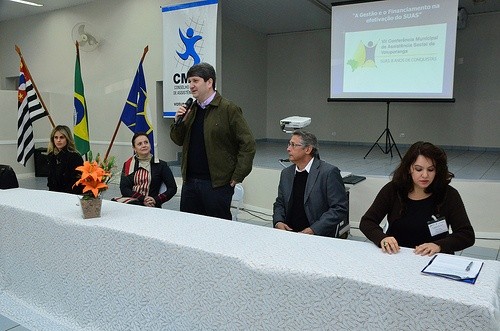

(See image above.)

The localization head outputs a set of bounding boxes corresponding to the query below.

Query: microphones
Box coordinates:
[173,98,193,126]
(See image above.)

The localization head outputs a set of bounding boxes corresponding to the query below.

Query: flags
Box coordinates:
[103,45,155,164]
[14,44,55,168]
[73,40,90,161]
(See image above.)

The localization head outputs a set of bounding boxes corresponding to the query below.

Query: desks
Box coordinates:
[0,188,500,331]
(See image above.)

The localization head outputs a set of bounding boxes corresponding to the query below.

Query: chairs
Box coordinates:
[0,165,19,190]
[230,184,245,222]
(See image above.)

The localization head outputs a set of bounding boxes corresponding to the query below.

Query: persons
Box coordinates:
[170,63,256,221]
[273,130,349,237]
[359,141,475,257]
[111,132,177,208]
[47,125,85,195]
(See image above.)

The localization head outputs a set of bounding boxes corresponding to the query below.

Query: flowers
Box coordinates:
[71,150,118,200]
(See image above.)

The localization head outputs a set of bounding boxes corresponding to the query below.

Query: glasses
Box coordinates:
[288,143,303,149]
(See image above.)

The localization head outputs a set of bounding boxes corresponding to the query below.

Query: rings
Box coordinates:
[383,240,389,246]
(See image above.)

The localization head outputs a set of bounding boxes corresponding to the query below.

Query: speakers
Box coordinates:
[34,148,49,177]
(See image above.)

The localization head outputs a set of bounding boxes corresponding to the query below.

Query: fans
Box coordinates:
[72,22,104,53]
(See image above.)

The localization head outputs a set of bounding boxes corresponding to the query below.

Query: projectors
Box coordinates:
[280,115,311,128]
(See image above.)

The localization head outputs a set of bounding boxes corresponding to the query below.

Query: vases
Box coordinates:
[80,199,103,219]
